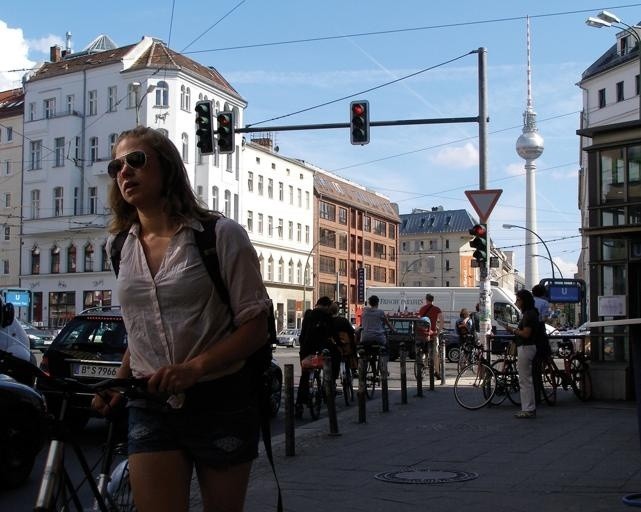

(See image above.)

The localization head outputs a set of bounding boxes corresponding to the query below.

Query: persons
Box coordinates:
[87,125,278,510]
[530,282,551,405]
[502,288,544,421]
[293,291,481,420]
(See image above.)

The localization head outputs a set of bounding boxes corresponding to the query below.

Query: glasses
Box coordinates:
[106,149,156,180]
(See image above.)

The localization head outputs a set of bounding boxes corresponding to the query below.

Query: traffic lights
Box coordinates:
[195,101,216,154]
[217,111,234,152]
[470,224,488,262]
[350,100,369,145]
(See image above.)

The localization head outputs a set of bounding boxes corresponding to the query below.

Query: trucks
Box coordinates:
[367,287,561,357]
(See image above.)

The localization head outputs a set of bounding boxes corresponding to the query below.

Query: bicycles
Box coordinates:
[1,349,152,512]
[454,340,593,410]
[458,338,483,375]
[414,343,441,379]
[307,342,381,420]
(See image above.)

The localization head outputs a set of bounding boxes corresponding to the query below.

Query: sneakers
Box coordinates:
[512,409,537,419]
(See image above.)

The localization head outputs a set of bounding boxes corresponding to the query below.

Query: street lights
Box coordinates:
[585,11,641,42]
[502,224,565,278]
[399,256,436,287]
[303,231,347,315]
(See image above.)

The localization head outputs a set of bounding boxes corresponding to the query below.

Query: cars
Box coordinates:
[277,329,301,347]
[387,316,461,362]
[548,327,589,358]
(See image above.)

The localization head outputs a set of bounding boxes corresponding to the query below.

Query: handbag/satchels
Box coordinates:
[255,362,282,418]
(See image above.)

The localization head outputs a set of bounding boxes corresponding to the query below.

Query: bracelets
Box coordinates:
[512,328,517,334]
[517,329,521,336]
